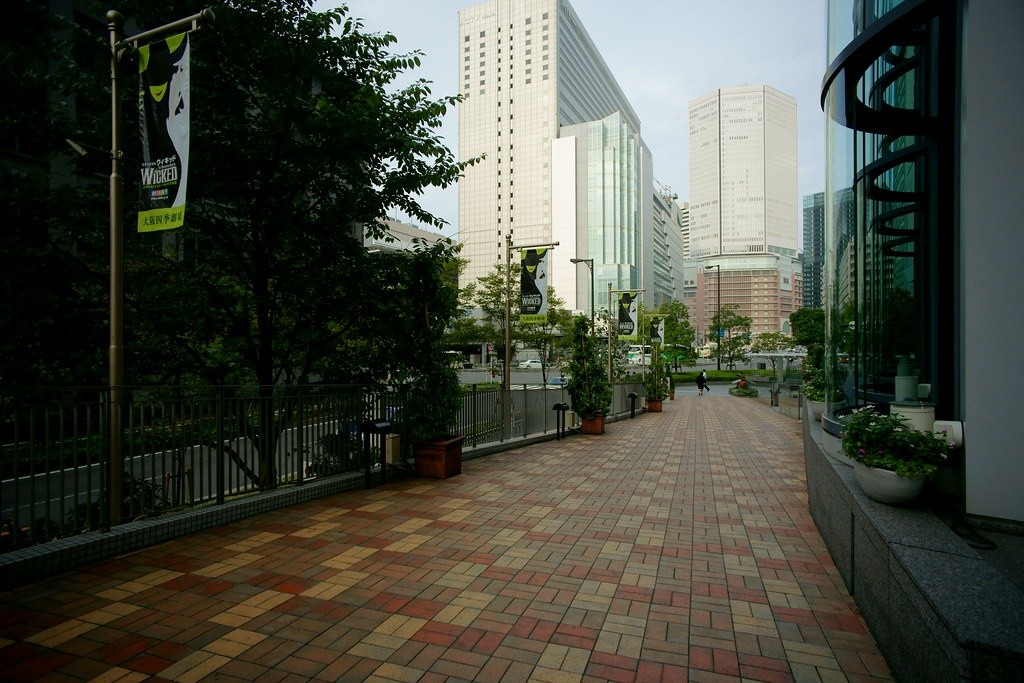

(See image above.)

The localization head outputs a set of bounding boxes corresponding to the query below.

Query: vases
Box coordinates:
[808,399,846,421]
[852,459,928,504]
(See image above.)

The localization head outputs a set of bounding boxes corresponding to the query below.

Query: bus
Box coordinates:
[628,344,689,366]
[442,351,465,369]
[699,346,715,359]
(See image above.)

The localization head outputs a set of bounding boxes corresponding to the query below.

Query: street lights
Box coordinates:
[705,265,721,371]
[571,259,594,335]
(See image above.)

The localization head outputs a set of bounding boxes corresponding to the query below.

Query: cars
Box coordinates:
[464,360,474,369]
[546,377,571,389]
[519,360,551,369]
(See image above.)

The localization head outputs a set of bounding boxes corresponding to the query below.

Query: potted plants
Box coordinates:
[640,351,672,412]
[557,312,615,435]
[390,311,476,479]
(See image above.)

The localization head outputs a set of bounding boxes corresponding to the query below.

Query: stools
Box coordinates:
[933,420,963,447]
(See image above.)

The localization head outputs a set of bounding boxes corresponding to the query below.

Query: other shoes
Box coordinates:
[699,393,700,396]
[707,388,710,392]
[701,393,703,395]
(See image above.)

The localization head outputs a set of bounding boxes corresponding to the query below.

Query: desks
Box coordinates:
[891,403,936,434]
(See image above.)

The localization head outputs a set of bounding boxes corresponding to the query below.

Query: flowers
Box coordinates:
[801,376,847,403]
[838,404,957,480]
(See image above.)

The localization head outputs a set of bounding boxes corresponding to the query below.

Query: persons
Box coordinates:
[696,369,710,396]
[736,372,743,381]
